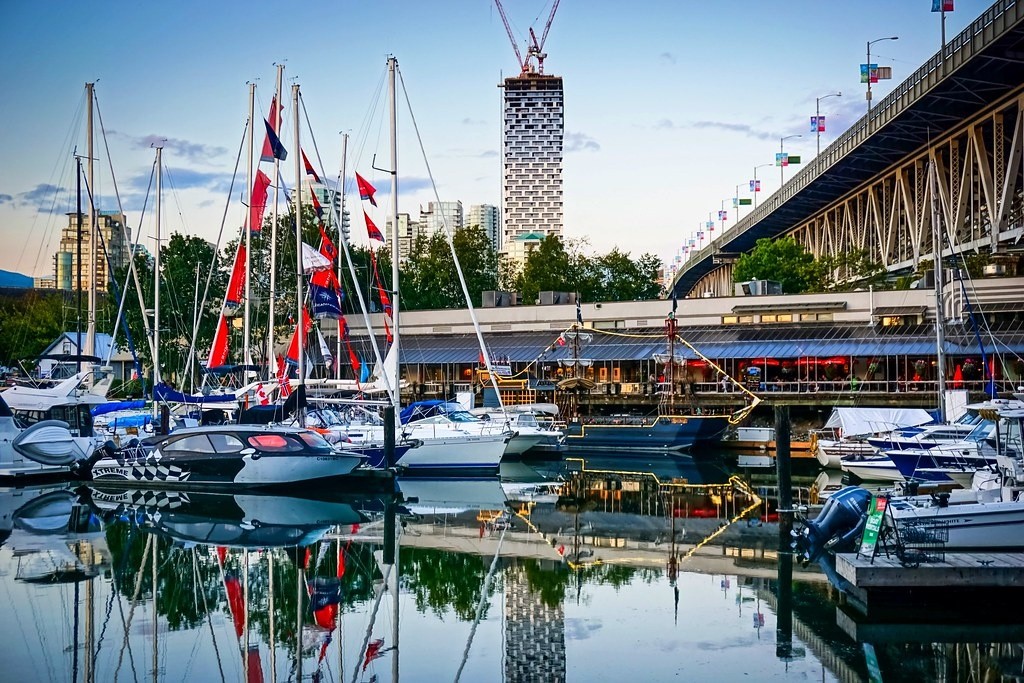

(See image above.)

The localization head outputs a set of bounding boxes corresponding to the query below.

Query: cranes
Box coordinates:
[492,0,560,78]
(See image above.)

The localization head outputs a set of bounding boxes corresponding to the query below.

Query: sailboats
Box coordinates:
[0,51,1023,570]
[0,476,557,683]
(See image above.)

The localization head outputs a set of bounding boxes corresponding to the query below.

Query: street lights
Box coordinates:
[754,164,774,209]
[816,92,842,176]
[780,135,802,204]
[866,35,900,135]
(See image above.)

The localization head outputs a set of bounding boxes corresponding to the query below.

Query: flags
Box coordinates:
[217,522,385,683]
[207,97,395,403]
